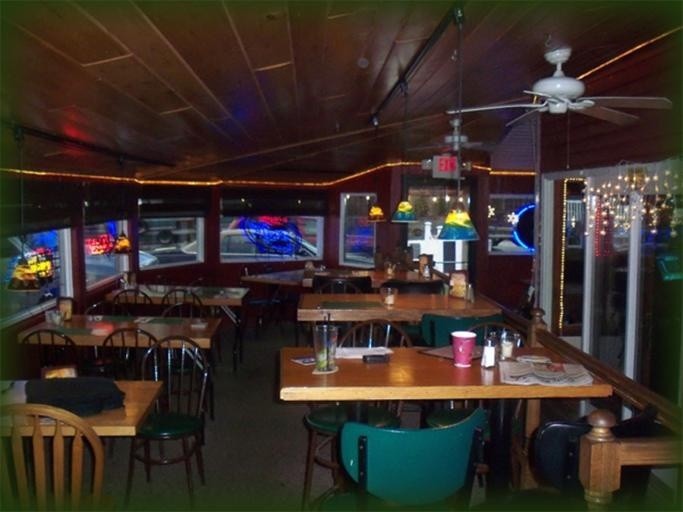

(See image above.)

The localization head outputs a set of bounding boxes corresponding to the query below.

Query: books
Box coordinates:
[498,359,594,388]
[335,346,394,359]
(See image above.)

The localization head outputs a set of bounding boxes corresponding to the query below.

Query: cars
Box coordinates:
[152,231,375,262]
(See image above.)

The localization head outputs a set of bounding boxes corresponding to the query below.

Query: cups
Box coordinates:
[56,297,73,322]
[44,310,66,336]
[450,331,477,367]
[312,324,338,372]
[384,262,393,274]
[379,287,397,305]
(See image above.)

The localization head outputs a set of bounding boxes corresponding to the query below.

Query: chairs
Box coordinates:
[427,323,527,487]
[242,264,282,328]
[162,303,211,319]
[113,289,155,318]
[315,407,491,512]
[302,317,412,511]
[16,328,84,381]
[0,402,106,512]
[161,289,205,315]
[514,404,660,512]
[423,313,507,433]
[84,300,134,317]
[124,336,210,511]
[101,328,163,381]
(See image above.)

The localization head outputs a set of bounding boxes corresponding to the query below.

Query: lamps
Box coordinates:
[436,198,481,242]
[112,151,132,255]
[8,126,42,294]
[391,80,421,223]
[366,117,387,222]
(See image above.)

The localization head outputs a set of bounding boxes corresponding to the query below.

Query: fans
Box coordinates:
[445,47,672,128]
[409,8,508,154]
